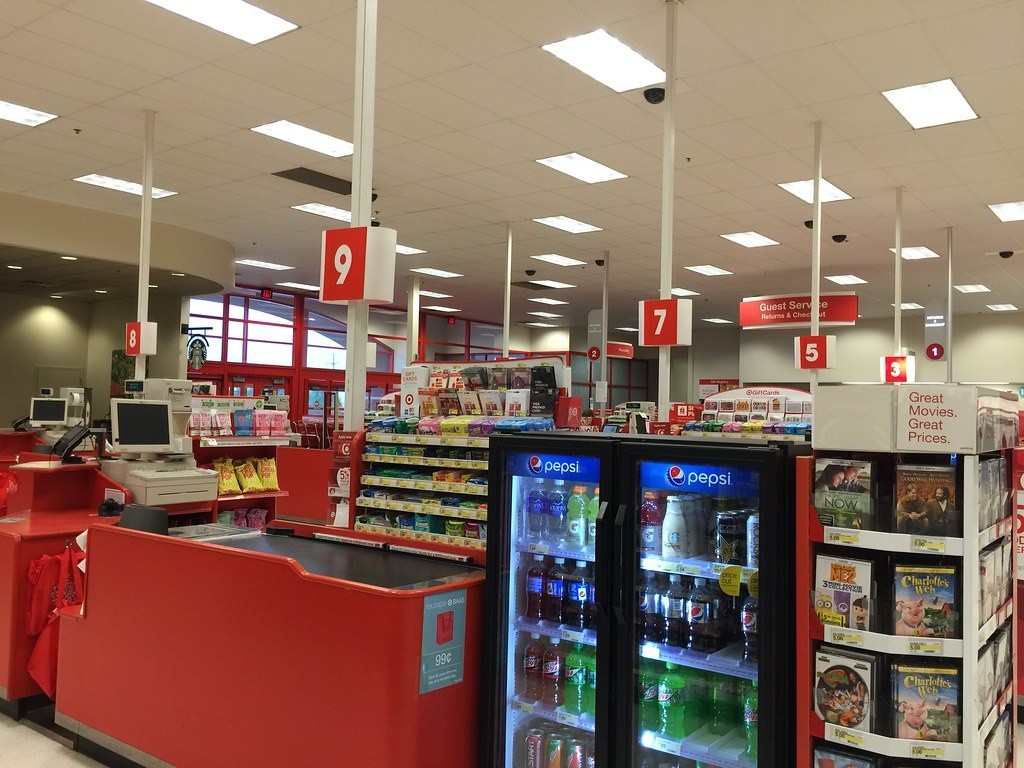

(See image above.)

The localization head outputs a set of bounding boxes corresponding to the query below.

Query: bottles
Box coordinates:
[589,576,596,629]
[661,493,711,559]
[542,638,566,706]
[566,485,590,546]
[589,487,600,544]
[547,557,570,623]
[640,488,682,556]
[737,676,758,763]
[524,554,548,620]
[524,633,546,700]
[665,575,689,648]
[529,478,548,544]
[742,595,759,663]
[686,577,746,655]
[569,561,589,628]
[699,669,735,736]
[565,643,597,716]
[639,656,701,740]
[547,480,568,543]
[639,571,665,642]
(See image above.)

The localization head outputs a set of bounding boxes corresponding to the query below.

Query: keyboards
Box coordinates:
[127,468,216,481]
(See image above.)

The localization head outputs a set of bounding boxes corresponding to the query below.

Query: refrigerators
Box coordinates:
[477,432,813,768]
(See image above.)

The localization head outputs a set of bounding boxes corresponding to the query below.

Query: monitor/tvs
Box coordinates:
[30,398,68,425]
[60,425,87,445]
[111,398,173,454]
[602,425,618,432]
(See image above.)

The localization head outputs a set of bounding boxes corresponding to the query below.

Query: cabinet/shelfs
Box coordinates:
[181,357,1024,768]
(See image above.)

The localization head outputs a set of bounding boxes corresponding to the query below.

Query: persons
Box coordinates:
[815,464,869,526]
[895,482,957,537]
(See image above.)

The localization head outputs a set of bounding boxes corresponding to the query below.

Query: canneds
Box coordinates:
[714,508,760,570]
[523,721,595,768]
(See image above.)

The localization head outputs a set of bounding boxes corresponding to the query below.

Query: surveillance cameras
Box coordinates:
[595,260,604,266]
[805,220,813,229]
[526,270,536,276]
[999,252,1014,258]
[372,194,378,202]
[832,235,847,243]
[644,88,665,105]
[371,221,380,228]
